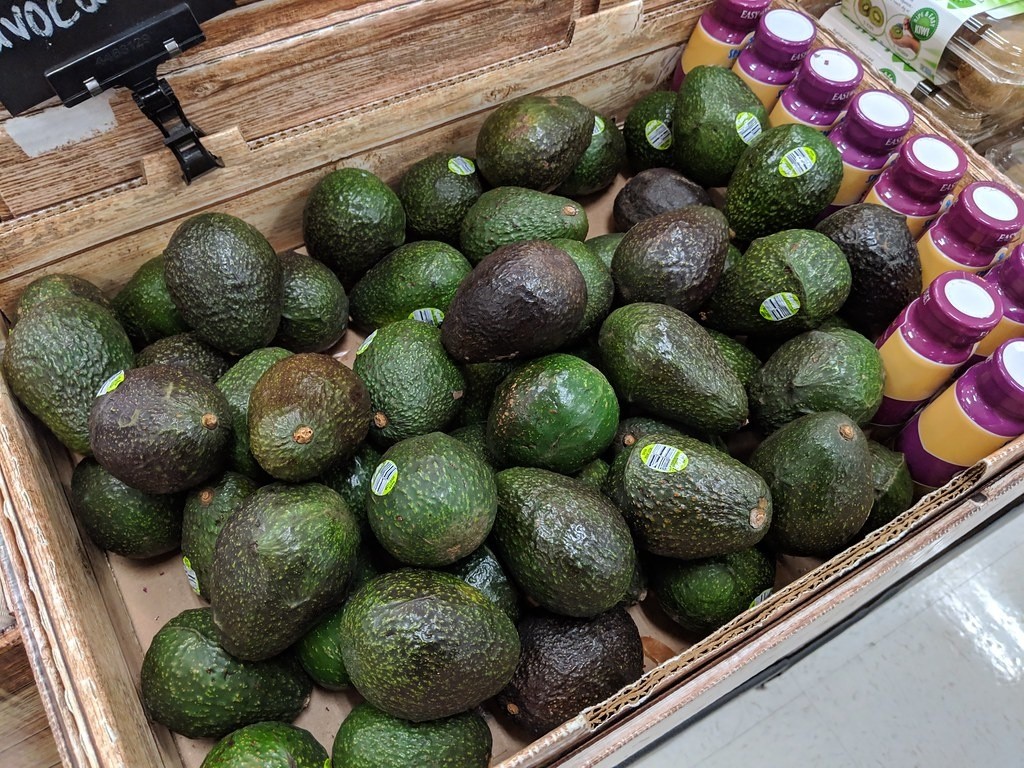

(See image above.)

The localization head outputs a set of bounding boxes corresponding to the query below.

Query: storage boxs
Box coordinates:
[0,1,1024,768]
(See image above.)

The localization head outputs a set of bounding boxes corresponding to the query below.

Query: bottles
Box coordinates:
[894,337,1024,490]
[916,180,1024,358]
[861,134,968,241]
[731,9,817,116]
[768,47,864,137]
[825,89,914,210]
[671,0,770,94]
[864,270,1004,425]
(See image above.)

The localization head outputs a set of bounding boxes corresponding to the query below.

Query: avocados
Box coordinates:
[2,65,912,768]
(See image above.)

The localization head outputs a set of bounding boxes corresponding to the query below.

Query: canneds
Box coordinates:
[669,0,1024,487]
